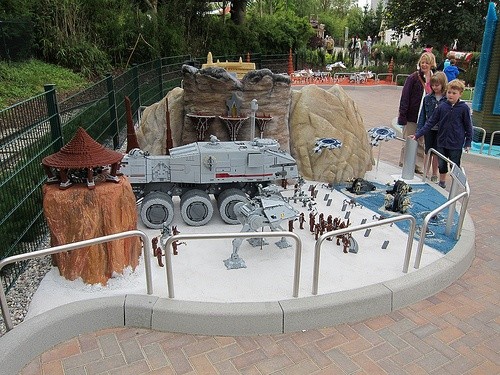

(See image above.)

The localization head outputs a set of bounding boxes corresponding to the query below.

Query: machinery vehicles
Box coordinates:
[104,99,300,229]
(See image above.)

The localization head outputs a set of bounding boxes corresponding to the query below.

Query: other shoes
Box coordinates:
[440,183,445,188]
[416,169,423,173]
[432,176,437,183]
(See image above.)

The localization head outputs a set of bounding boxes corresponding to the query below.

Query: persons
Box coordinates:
[293,175,353,254]
[398,52,473,191]
[321,34,386,67]
[420,43,467,82]
[151,220,185,267]
